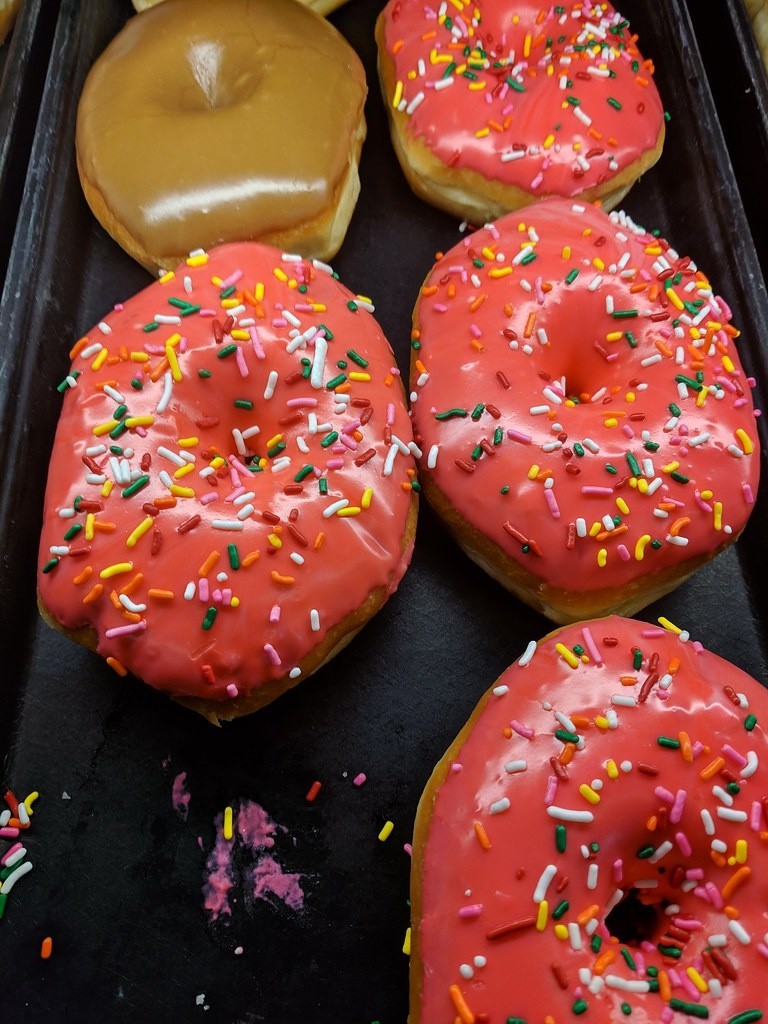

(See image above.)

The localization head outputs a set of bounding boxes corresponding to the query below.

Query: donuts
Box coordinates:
[36,1,768,1024]
